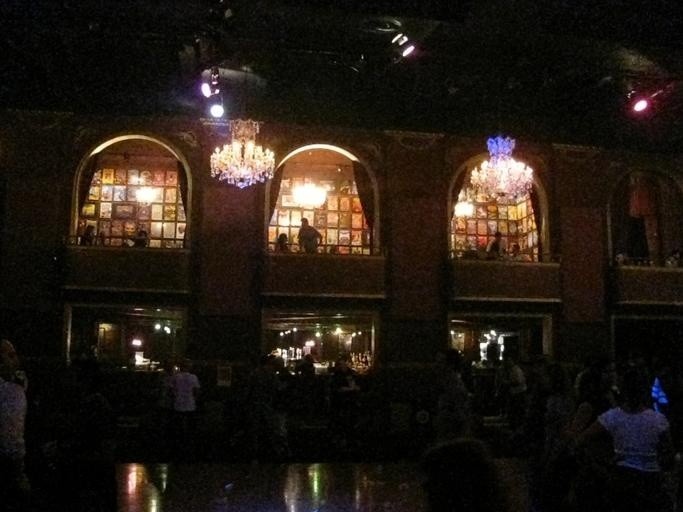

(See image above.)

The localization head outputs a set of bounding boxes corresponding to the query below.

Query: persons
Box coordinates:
[484,232,505,258]
[0,335,683,512]
[276,233,289,252]
[484,243,500,261]
[296,218,322,254]
[79,225,94,247]
[129,230,147,249]
[506,244,520,261]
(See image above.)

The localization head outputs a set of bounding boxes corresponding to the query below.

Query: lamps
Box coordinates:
[377,21,416,58]
[200,66,224,119]
[627,90,649,112]
